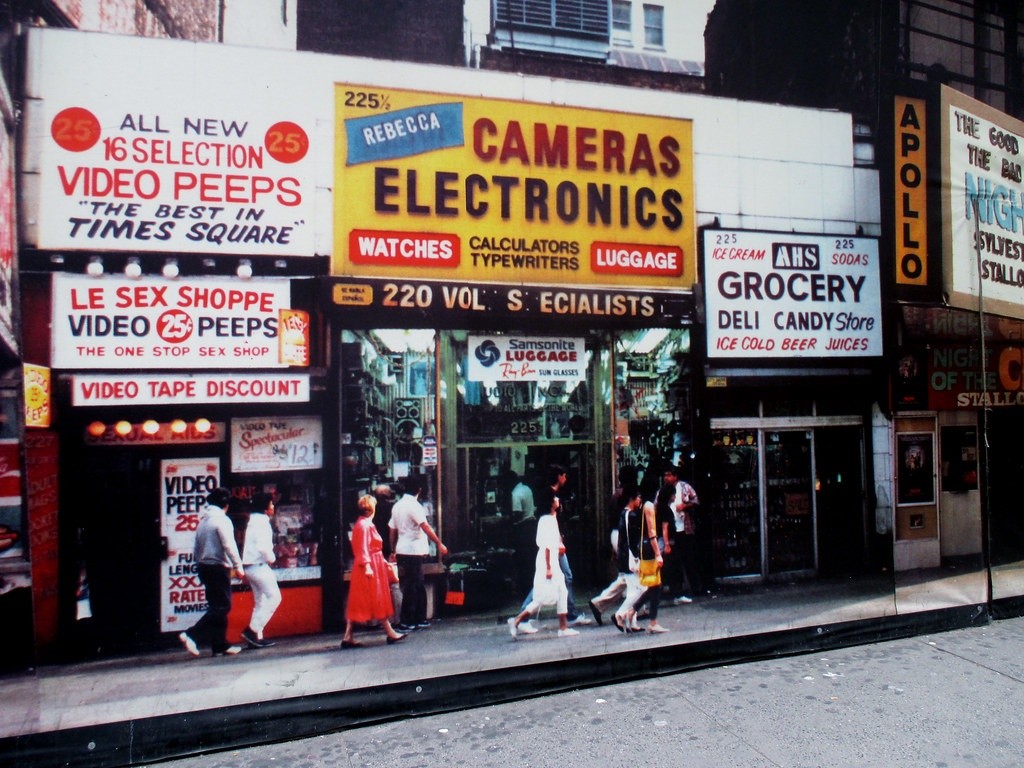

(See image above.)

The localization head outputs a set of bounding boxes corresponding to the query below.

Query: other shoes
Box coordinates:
[518,621,539,633]
[630,624,645,632]
[558,629,579,637]
[567,615,592,628]
[508,618,518,641]
[340,639,364,648]
[399,620,431,630]
[210,645,242,656]
[179,632,201,656]
[387,634,408,645]
[611,614,624,632]
[589,601,603,626]
[647,623,670,634]
[674,595,693,605]
[241,626,274,648]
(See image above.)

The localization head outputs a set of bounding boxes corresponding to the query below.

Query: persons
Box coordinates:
[179,488,245,657]
[507,465,699,640]
[341,495,409,648]
[240,493,282,647]
[389,476,448,633]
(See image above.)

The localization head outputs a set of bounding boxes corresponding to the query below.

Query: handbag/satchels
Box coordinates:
[639,559,662,587]
[628,550,641,573]
